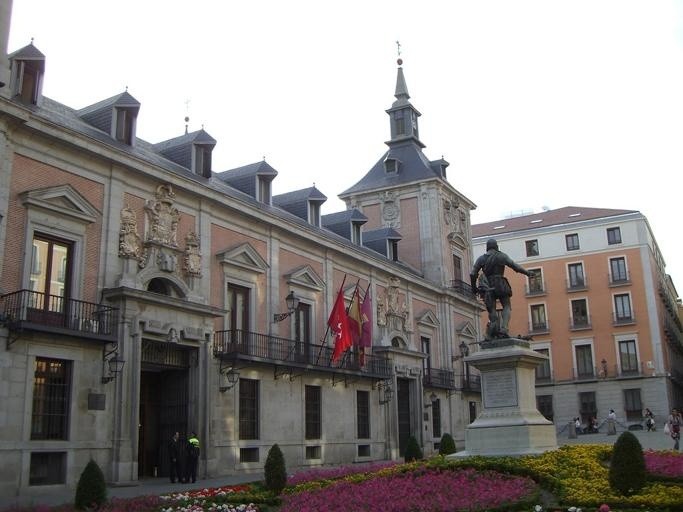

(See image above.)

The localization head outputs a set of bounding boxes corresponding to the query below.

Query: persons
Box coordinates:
[470,238,536,339]
[606,407,617,435]
[575,416,583,435]
[666,408,683,452]
[590,416,599,434]
[643,408,656,432]
[167,430,201,485]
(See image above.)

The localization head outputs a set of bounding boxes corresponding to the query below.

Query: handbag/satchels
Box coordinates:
[673,425,680,433]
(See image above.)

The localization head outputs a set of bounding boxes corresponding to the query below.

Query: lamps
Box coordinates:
[102,350,126,386]
[219,364,241,392]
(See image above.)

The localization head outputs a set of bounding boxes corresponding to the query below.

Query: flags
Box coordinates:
[326,285,376,369]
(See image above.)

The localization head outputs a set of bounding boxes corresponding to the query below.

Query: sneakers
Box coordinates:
[170,479,197,484]
